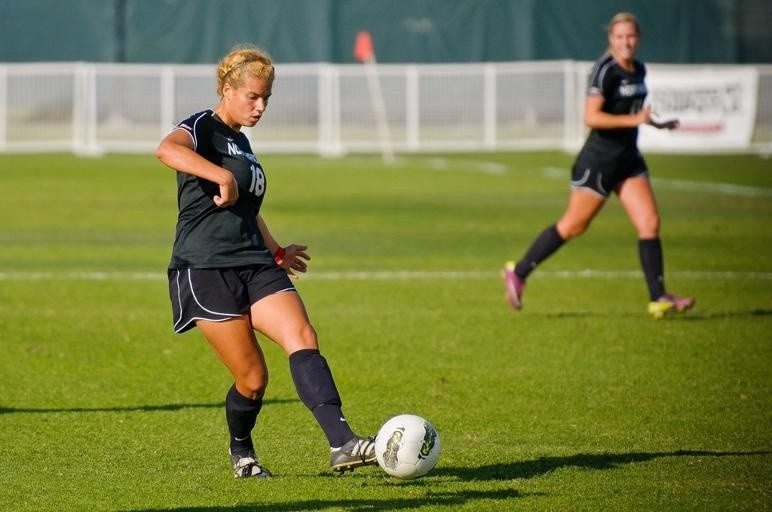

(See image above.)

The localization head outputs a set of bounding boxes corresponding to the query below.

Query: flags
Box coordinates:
[354,30,373,62]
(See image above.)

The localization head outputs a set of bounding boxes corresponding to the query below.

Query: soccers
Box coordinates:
[376,414,440,479]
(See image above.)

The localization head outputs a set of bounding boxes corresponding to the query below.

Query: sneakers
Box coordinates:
[497,260,526,311]
[326,435,378,473]
[226,446,272,480]
[646,294,697,319]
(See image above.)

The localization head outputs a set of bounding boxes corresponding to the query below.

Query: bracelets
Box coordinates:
[272,246,287,263]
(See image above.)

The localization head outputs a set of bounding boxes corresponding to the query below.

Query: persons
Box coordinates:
[494,12,695,321]
[154,42,375,477]
